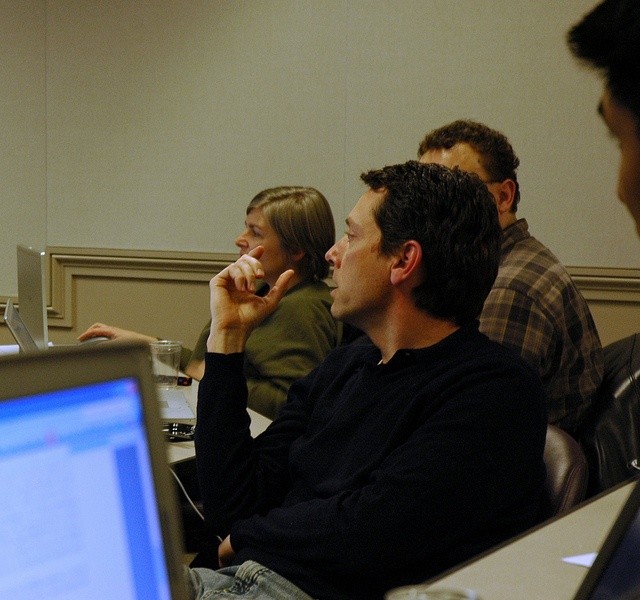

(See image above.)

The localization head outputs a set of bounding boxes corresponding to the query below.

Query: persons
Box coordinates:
[416,119,606,424]
[193,159,549,599]
[565,1,639,245]
[77,186,335,421]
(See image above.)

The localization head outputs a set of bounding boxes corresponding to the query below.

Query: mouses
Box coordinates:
[78,337,108,346]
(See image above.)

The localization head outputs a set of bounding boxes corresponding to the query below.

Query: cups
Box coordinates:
[150,340,182,388]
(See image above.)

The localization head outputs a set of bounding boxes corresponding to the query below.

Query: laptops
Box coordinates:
[4,299,199,441]
[15,243,195,387]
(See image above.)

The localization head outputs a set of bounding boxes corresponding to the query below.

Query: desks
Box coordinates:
[3,339,275,468]
[407,475,637,598]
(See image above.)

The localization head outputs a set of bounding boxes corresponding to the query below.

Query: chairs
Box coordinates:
[529,423,588,530]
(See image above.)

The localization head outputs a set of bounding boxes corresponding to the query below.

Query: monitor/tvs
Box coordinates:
[1,341,189,600]
